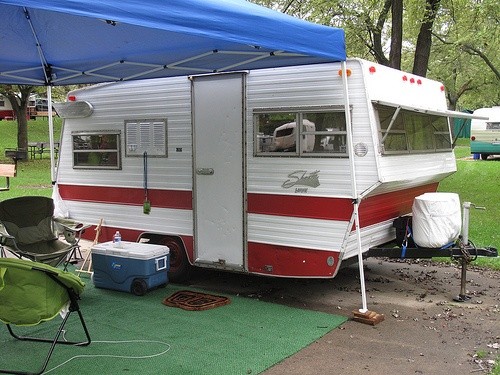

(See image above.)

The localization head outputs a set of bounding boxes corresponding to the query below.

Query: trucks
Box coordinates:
[51,58,489,284]
[470,105,500,160]
[0,91,37,121]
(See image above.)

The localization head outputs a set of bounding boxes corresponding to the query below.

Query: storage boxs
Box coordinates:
[90,241,170,297]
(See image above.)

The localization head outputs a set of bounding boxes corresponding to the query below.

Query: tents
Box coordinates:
[0,0,368,314]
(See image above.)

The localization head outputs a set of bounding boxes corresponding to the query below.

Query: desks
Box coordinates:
[28,142,37,159]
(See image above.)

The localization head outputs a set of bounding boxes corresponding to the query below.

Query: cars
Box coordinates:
[36,98,55,111]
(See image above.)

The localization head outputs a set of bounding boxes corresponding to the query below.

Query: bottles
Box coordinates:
[112,231,122,248]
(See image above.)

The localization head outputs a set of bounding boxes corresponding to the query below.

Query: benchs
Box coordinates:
[37,141,60,160]
[31,111,56,121]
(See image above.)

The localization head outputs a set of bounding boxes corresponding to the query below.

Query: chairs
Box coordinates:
[0,258,93,375]
[0,196,91,272]
